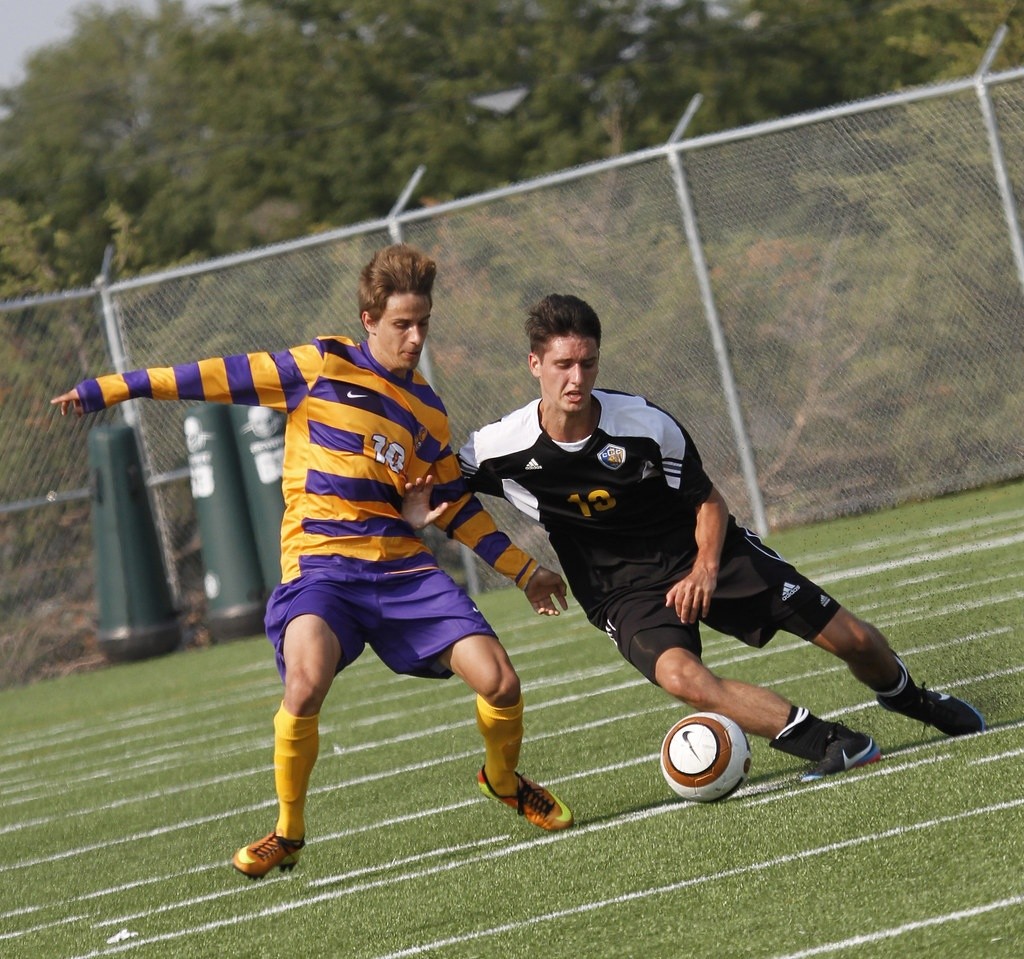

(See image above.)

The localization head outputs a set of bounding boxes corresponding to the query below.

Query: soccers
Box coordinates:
[660,711,752,804]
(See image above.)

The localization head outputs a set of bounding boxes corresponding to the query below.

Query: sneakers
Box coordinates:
[476,762,574,832]
[232,829,306,881]
[798,732,882,783]
[875,684,983,736]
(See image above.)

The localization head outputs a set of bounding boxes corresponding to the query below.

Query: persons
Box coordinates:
[401,294,985,782]
[49,243,573,882]
